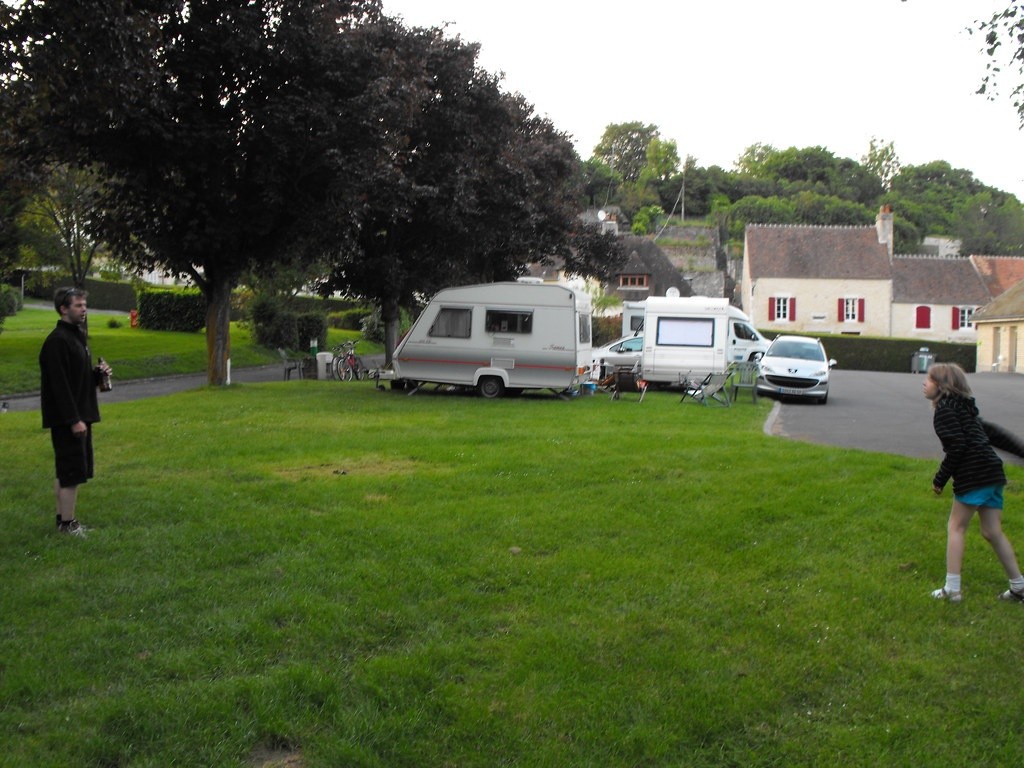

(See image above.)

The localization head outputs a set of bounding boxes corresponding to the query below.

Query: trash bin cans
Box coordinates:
[316,352,336,379]
[912,352,937,374]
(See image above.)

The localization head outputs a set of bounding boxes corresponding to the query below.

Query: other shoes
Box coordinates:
[56,518,88,540]
[56,514,95,533]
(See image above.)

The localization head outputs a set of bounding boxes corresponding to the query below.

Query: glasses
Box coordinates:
[62,287,78,304]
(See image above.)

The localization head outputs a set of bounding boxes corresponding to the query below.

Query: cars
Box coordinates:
[592,336,643,367]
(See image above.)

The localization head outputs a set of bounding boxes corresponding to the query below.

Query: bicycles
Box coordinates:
[330,339,366,382]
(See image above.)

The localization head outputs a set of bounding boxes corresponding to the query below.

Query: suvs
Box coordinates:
[755,334,837,405]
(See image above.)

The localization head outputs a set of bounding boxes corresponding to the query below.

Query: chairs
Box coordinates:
[729,362,760,404]
[678,370,731,409]
[277,348,302,381]
[609,371,649,403]
[576,366,622,393]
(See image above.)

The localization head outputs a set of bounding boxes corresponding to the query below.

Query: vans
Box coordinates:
[622,301,772,364]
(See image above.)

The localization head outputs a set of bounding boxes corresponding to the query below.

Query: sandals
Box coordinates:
[929,588,962,604]
[997,588,1024,603]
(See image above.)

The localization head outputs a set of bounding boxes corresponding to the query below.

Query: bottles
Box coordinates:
[97,357,112,392]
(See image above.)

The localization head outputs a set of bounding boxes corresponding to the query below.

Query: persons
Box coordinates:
[923,362,1024,602]
[39,286,113,538]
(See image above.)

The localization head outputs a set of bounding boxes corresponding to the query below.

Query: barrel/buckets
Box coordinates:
[581,383,595,394]
